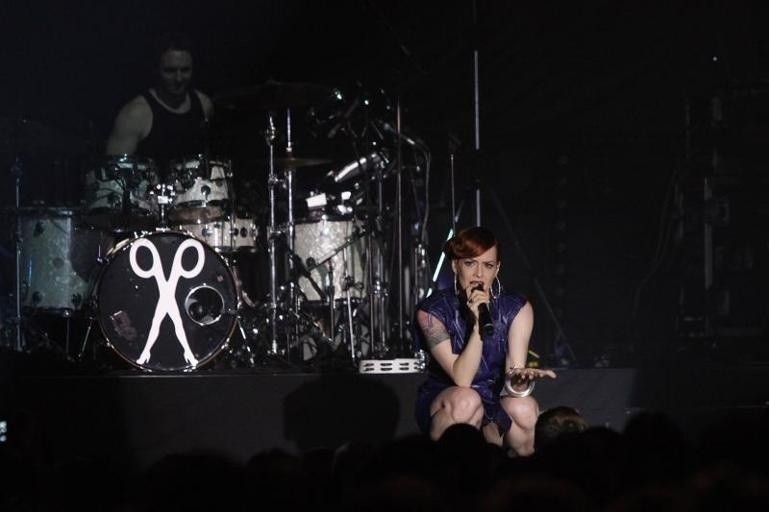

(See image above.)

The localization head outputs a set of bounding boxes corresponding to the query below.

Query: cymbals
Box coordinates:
[255,158,334,169]
[205,81,337,112]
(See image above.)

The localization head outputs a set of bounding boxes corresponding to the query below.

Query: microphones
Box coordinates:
[471,283,495,337]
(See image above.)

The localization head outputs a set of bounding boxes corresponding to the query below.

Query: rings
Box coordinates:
[468,297,474,304]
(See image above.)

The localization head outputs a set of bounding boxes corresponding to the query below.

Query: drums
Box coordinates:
[80,155,162,226]
[85,229,241,377]
[270,217,369,311]
[18,207,114,314]
[182,209,256,252]
[163,156,231,222]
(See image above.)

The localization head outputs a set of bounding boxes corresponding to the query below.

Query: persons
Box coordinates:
[102,34,219,170]
[0,403,769,512]
[412,226,559,460]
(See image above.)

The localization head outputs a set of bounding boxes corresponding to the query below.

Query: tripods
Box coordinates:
[424,149,578,364]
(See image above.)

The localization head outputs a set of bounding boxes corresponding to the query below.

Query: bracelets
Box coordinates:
[503,374,536,398]
